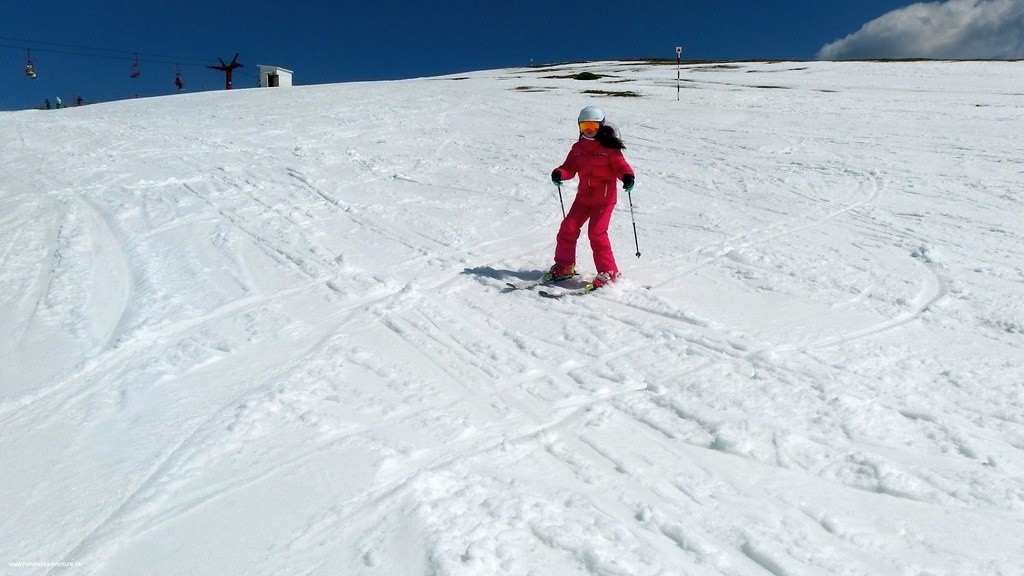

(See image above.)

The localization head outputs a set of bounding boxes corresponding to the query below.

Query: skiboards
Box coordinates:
[505,270,623,299]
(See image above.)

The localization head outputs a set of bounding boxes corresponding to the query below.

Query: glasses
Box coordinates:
[579,122,599,130]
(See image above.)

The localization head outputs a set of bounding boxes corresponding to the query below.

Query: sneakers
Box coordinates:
[594,272,619,287]
[550,266,574,277]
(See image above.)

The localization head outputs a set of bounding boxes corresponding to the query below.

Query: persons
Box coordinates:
[27,59,37,79]
[44,99,51,110]
[175,78,182,89]
[545,106,635,287]
[56,97,61,109]
[77,96,84,106]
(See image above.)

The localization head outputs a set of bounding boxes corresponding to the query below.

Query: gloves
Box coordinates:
[623,174,635,192]
[551,171,563,186]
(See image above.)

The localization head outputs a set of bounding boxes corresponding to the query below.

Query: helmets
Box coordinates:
[578,106,605,126]
